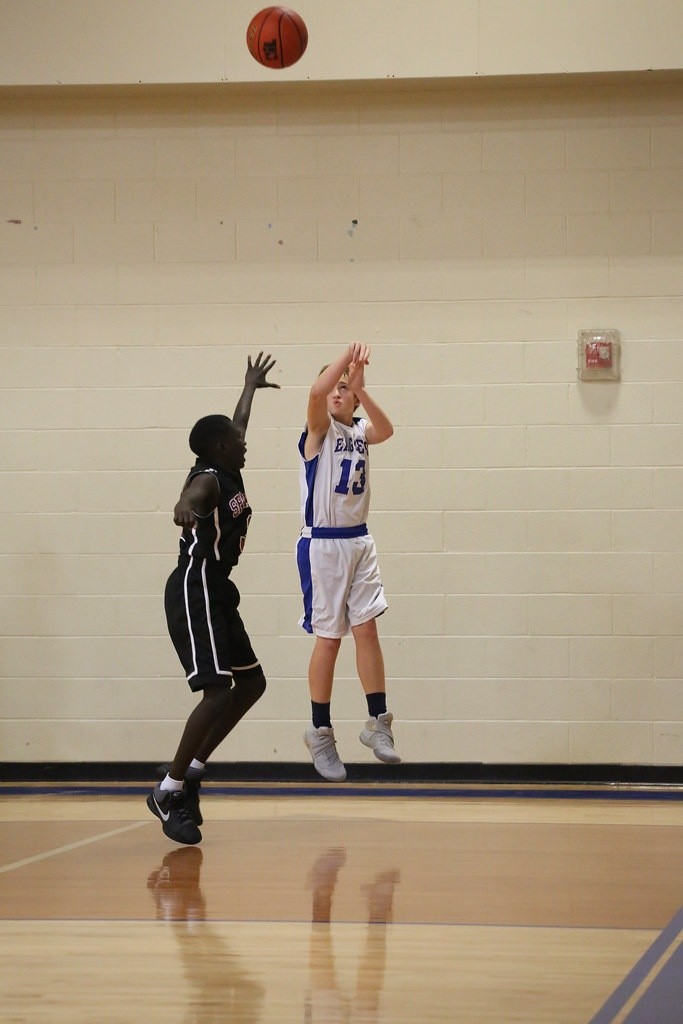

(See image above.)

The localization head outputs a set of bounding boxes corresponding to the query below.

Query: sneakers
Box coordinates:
[360,710,402,764]
[304,721,347,782]
[146,768,203,845]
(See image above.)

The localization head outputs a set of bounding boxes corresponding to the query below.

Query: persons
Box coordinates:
[298,343,401,781]
[146,352,282,845]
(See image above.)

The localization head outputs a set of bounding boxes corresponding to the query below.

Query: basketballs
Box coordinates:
[245,5,309,69]
